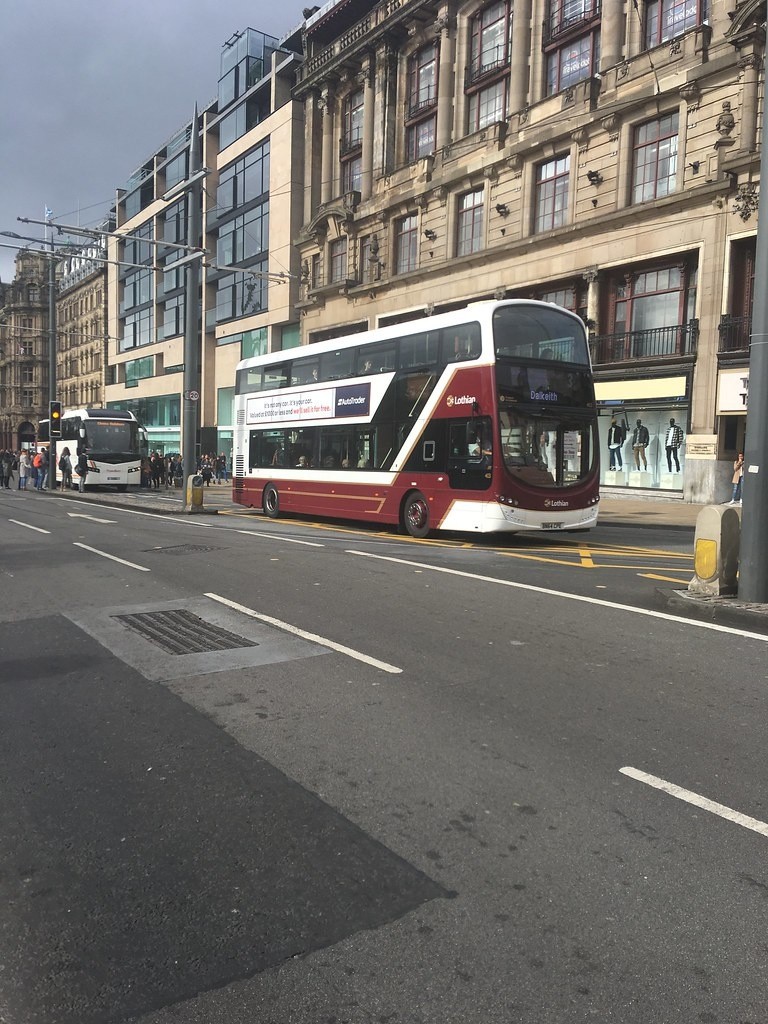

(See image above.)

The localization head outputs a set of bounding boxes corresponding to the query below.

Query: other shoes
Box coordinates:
[60,489,63,491]
[5,487,11,489]
[63,489,70,492]
[618,467,622,472]
[0,487,3,490]
[728,500,735,505]
[740,499,742,504]
[611,467,616,470]
[79,491,88,493]
[37,488,47,492]
[23,488,31,492]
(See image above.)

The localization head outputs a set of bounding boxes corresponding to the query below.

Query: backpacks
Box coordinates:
[59,455,68,470]
[33,454,42,467]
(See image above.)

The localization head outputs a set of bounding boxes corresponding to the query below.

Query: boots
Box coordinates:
[20,488,24,491]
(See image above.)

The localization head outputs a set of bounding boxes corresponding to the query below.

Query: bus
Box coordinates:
[231,298,602,539]
[37,408,148,492]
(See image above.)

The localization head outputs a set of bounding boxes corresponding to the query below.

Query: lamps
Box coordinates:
[425,228,437,241]
[496,204,508,215]
[587,170,603,183]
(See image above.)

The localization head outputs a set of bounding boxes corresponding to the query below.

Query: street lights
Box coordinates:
[0,230,104,490]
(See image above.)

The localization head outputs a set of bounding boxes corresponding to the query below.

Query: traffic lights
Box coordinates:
[49,401,62,438]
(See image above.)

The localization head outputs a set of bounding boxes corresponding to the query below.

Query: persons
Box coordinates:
[60,447,72,492]
[632,419,649,471]
[728,451,744,505]
[540,431,549,466]
[358,357,377,375]
[85,437,96,450]
[141,451,229,489]
[665,417,683,472]
[540,348,553,360]
[608,419,624,471]
[0,446,50,491]
[307,368,319,383]
[79,447,89,493]
[468,424,493,457]
[262,443,371,468]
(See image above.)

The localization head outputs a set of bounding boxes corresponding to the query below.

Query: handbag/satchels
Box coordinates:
[75,465,86,476]
